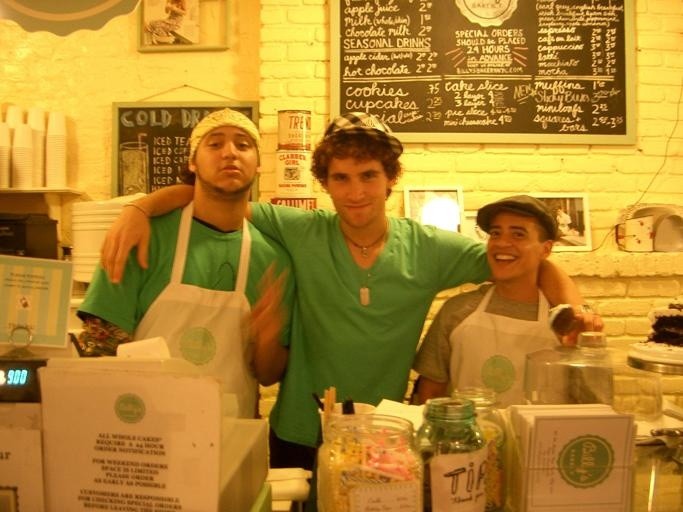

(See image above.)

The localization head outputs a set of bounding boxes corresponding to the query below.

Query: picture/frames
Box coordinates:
[524,191,595,253]
[402,183,466,238]
[135,0,233,54]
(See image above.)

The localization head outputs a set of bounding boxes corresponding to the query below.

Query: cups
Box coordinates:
[318,402,375,442]
[0,104,71,189]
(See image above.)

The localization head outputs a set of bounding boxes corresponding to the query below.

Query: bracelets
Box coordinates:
[575,304,594,313]
[122,203,150,217]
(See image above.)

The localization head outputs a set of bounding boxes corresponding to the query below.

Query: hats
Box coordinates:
[321,111,403,161]
[188,107,263,167]
[475,194,560,241]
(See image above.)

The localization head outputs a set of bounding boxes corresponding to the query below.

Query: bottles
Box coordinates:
[414,384,509,512]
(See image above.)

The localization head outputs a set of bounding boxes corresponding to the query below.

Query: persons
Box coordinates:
[76,108,297,419]
[99,111,603,512]
[410,193,566,406]
[554,206,572,242]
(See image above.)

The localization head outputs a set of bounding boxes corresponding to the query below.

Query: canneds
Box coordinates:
[268,109,316,211]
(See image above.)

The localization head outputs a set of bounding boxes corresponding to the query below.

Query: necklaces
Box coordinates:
[354,257,377,306]
[342,221,388,258]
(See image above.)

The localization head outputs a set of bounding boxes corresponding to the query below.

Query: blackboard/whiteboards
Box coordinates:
[111,101,260,202]
[328,0,638,147]
